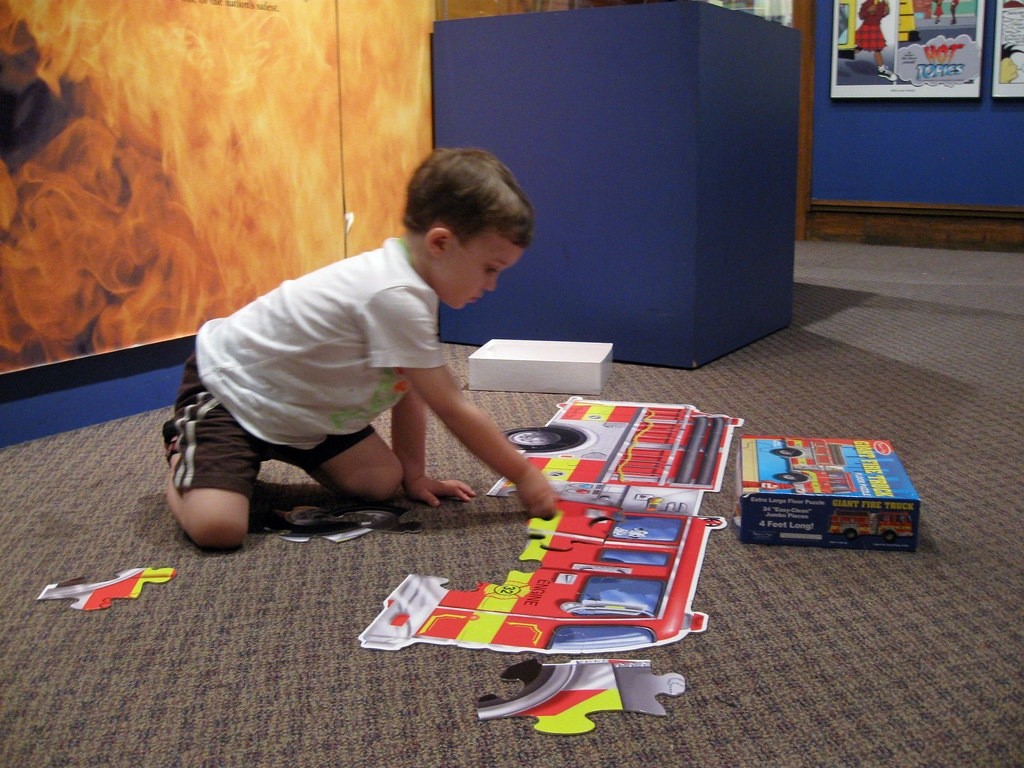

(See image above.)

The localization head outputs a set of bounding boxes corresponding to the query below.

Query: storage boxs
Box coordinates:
[731,435,921,552]
[468,339,613,395]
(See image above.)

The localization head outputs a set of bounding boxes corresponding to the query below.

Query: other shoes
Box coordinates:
[162,420,180,459]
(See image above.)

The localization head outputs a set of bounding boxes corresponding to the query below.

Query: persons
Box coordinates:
[854,0,898,80]
[160,147,563,551]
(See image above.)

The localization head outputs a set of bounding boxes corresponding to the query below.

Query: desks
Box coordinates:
[433,1,803,369]
